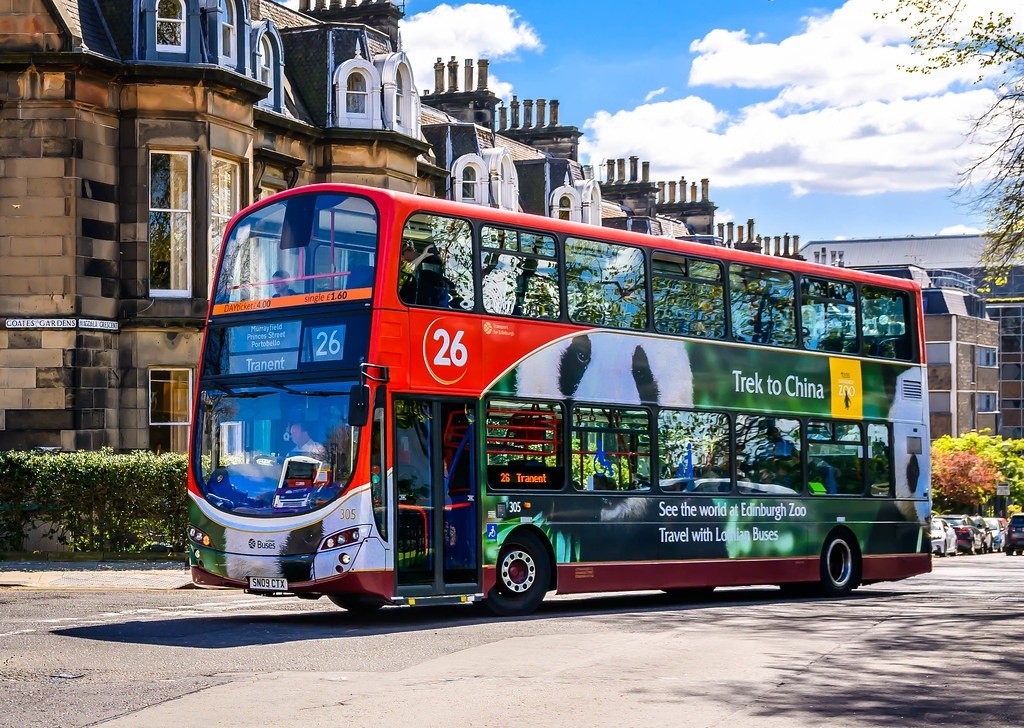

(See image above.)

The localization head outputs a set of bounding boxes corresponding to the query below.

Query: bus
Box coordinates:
[184,182,935,616]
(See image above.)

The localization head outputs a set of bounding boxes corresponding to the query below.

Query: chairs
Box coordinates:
[346,265,375,288]
[400,254,449,309]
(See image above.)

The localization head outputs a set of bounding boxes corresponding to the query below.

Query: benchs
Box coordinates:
[845,335,910,358]
[811,455,861,494]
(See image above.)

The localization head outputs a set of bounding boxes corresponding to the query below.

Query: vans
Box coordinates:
[1002,513,1024,556]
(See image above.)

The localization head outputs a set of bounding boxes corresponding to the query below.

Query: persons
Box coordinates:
[271,271,296,298]
[400,243,434,304]
[283,421,327,461]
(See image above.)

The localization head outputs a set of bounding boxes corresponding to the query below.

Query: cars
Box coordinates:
[929,514,1010,558]
